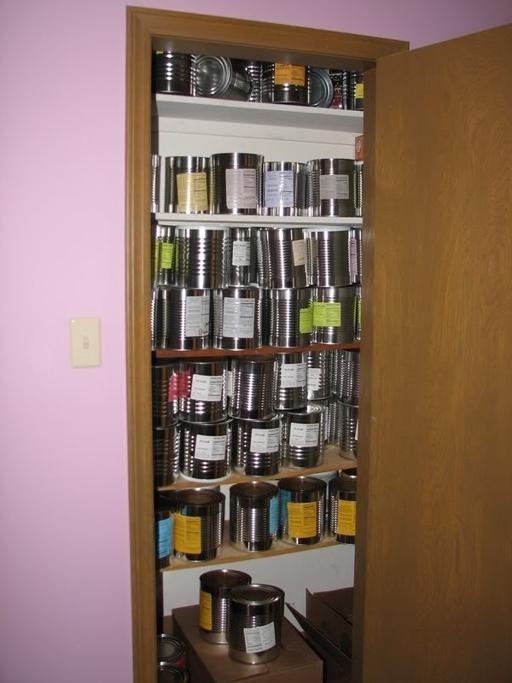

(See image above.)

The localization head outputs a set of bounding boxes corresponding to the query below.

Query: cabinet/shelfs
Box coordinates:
[122,4,511,683]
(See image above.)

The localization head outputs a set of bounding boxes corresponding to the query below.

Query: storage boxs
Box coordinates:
[170,600,324,683]
[285,581,352,668]
